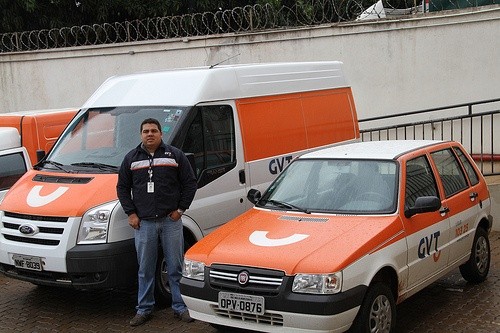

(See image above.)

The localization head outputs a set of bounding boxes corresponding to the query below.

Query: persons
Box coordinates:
[116,117,198,326]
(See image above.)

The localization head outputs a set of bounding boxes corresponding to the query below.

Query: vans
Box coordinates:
[0,59,359,307]
[0,107,118,214]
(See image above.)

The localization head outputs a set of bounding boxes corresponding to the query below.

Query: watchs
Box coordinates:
[176,207,183,215]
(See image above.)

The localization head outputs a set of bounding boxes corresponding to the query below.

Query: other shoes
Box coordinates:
[178,309,194,322]
[130,314,153,325]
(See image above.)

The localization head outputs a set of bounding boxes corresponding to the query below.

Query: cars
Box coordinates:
[178,139,494,332]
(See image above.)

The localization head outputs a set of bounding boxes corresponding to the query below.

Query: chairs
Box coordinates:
[319,164,453,219]
